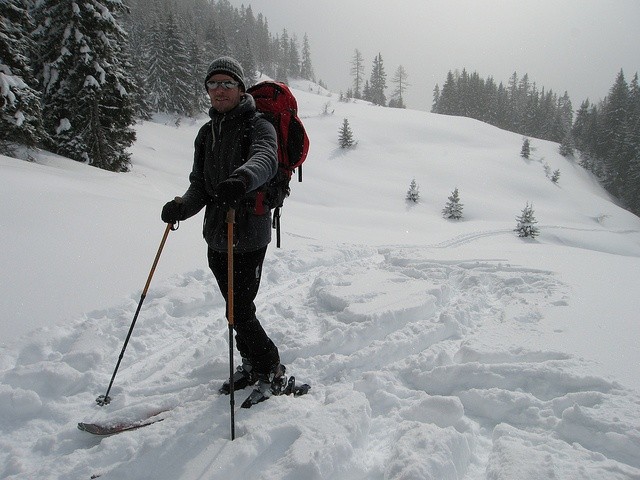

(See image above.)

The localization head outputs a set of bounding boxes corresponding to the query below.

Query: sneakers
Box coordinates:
[241,363,287,408]
[223,356,259,390]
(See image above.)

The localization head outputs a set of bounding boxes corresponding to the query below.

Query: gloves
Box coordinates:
[216,173,249,201]
[161,200,181,224]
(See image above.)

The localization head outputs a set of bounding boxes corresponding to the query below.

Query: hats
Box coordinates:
[205,56,246,94]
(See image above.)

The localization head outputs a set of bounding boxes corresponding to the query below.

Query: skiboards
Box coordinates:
[78,364,311,479]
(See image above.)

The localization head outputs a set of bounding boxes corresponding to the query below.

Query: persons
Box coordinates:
[161,56,292,406]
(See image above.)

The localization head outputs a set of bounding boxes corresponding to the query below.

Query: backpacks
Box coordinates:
[246,80,309,248]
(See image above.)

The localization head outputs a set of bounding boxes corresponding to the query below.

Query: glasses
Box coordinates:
[206,79,240,90]
